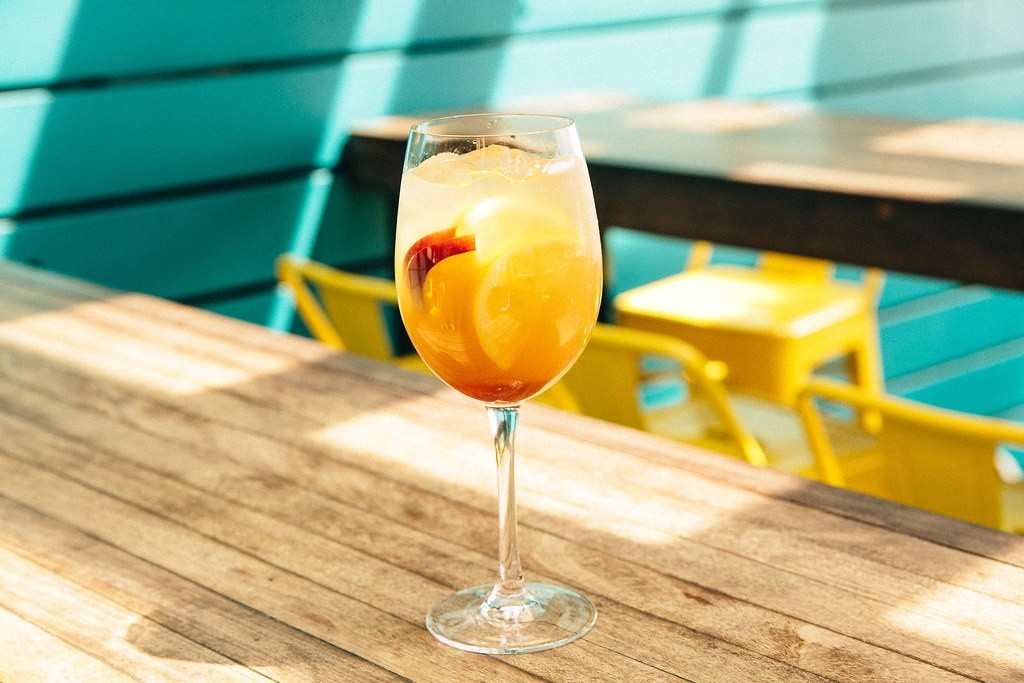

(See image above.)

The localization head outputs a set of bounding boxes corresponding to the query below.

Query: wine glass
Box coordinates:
[393,112,604,656]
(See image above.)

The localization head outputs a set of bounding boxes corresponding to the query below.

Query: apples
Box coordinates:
[403,227,478,314]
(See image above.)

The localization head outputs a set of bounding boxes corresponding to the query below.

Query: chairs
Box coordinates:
[274,241,1023,534]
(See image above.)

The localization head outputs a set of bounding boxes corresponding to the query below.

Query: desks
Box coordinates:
[335,97,1022,360]
[1,258,1024,683]
